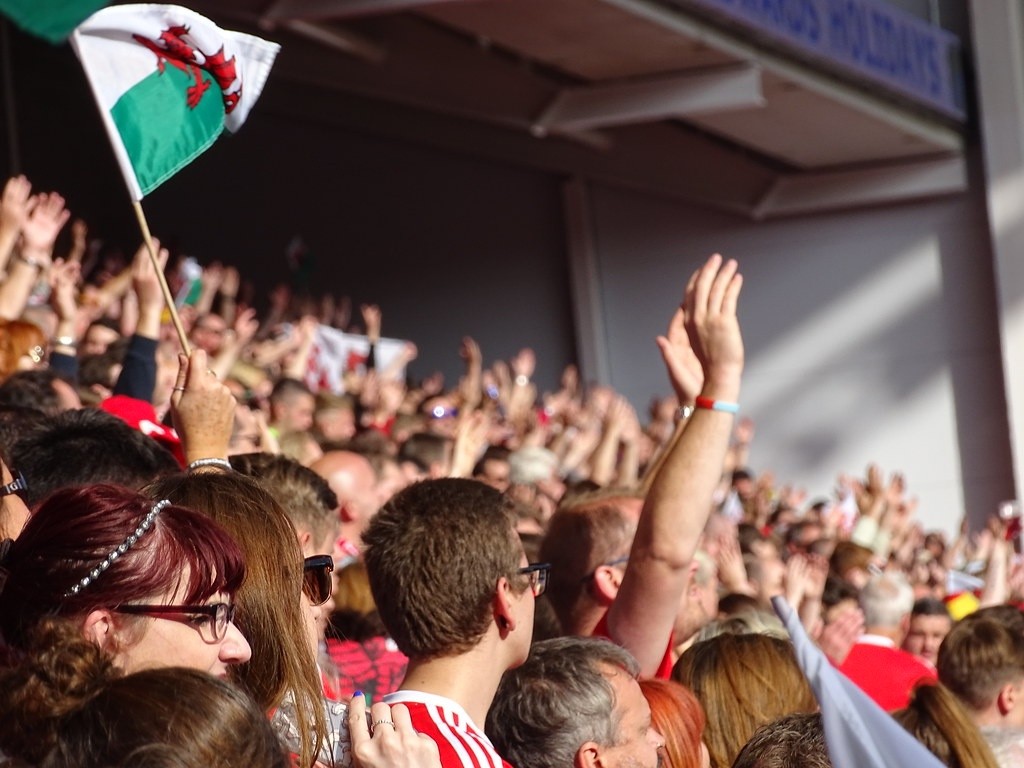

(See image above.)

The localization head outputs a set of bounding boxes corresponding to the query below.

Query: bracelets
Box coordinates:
[694,396,741,413]
[181,458,233,473]
[672,406,695,418]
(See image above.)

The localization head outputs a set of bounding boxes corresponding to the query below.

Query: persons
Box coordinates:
[0,173,1024,768]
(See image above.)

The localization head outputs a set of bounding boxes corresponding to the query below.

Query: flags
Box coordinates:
[0,0,282,200]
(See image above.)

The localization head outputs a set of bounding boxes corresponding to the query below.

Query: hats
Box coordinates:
[427,406,457,418]
[99,394,180,444]
[574,480,601,493]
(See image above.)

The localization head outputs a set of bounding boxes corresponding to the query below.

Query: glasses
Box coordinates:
[520,560,551,598]
[21,352,50,370]
[109,603,237,645]
[301,554,334,607]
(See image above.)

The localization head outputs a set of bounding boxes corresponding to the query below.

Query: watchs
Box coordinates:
[0,471,29,501]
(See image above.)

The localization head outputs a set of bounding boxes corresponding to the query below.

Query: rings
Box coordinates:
[171,387,185,391]
[206,368,217,377]
[370,720,398,732]
